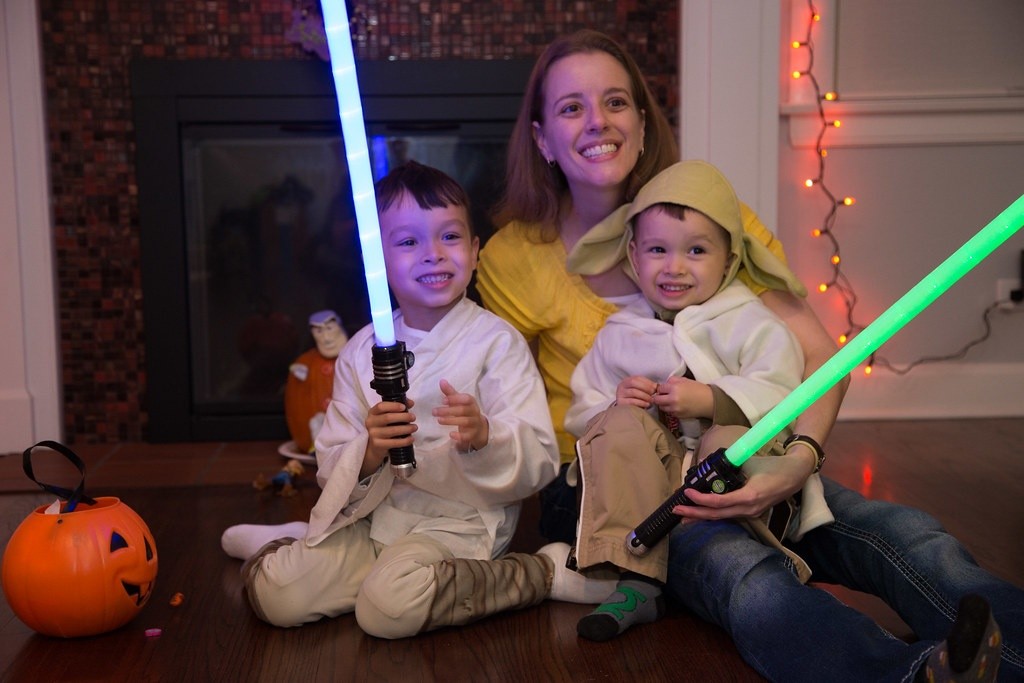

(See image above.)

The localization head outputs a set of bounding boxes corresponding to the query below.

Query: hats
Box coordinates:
[566,160,807,320]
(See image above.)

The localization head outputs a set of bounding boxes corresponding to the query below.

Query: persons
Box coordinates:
[564,161,806,641]
[473,30,1024,683]
[222,163,619,640]
[284,311,348,457]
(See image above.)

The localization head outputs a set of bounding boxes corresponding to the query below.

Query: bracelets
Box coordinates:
[783,433,826,474]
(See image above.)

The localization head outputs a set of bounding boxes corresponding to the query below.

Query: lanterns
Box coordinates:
[0,496,157,639]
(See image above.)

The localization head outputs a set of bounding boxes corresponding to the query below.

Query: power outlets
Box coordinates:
[997,277,1024,309]
